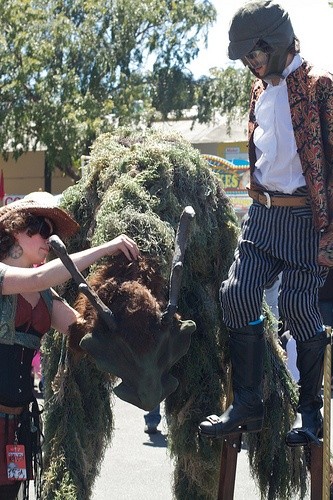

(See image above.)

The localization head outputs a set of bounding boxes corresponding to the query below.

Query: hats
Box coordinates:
[228,0,295,86]
[0,191,80,234]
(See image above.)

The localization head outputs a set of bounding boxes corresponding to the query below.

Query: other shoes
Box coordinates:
[145,425,162,436]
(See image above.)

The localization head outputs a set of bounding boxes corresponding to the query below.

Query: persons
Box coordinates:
[144,403,162,433]
[0,191,142,500]
[196,0,333,446]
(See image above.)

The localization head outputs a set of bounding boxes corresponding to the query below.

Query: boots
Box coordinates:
[200,318,265,438]
[285,331,327,447]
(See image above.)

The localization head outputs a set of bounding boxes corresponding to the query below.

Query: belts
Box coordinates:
[248,188,310,209]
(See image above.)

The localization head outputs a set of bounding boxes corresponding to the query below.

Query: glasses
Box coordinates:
[245,45,272,59]
[28,216,55,240]
